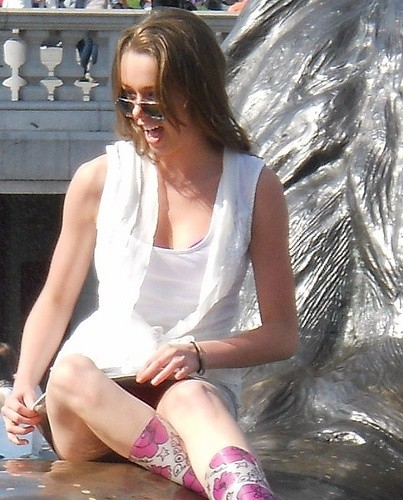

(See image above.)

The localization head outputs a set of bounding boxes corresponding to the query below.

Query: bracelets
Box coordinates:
[193,340,207,375]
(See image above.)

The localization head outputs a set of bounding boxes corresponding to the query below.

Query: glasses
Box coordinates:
[113,95,187,121]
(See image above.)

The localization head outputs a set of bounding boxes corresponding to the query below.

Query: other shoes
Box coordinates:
[202,445,278,500]
[126,413,208,499]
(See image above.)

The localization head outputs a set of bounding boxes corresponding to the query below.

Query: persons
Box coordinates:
[2,6,304,500]
[3,0,244,83]
